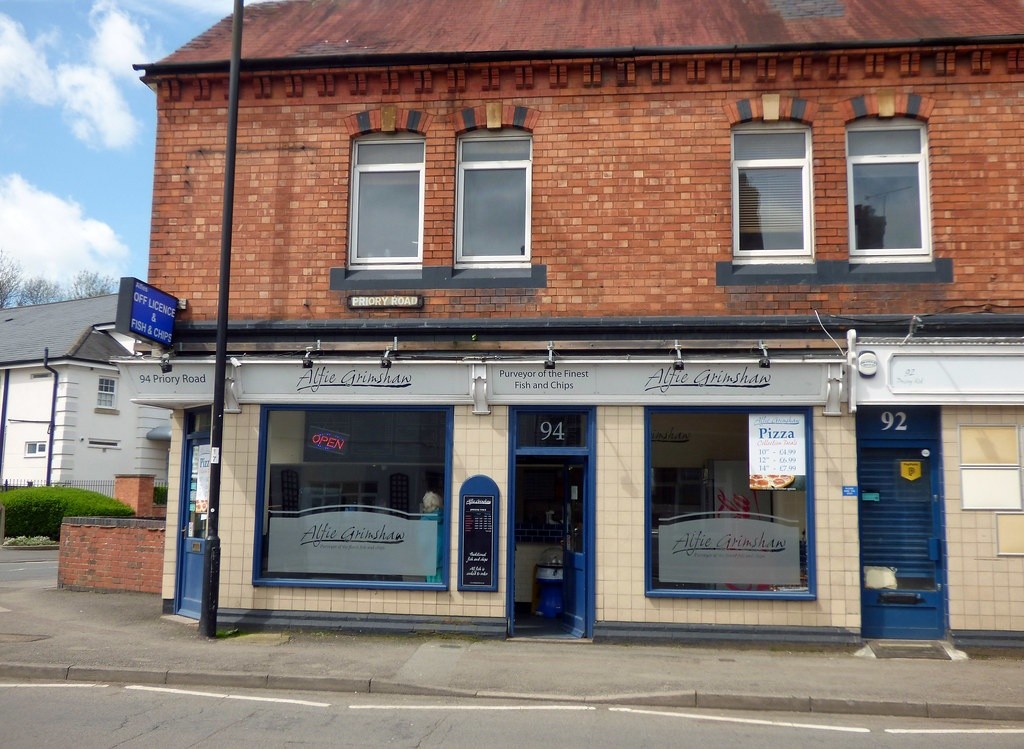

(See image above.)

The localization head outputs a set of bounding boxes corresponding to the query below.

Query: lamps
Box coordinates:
[544,341,557,371]
[302,339,320,368]
[758,340,772,368]
[380,337,397,370]
[160,349,174,374]
[673,339,685,371]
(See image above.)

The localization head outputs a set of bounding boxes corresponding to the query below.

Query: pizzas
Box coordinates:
[196,500,207,512]
[750,474,795,489]
